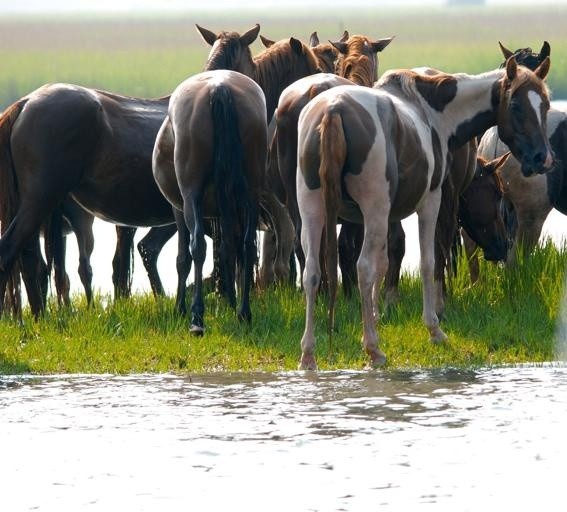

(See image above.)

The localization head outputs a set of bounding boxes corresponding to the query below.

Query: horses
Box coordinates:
[1,21,567,371]
